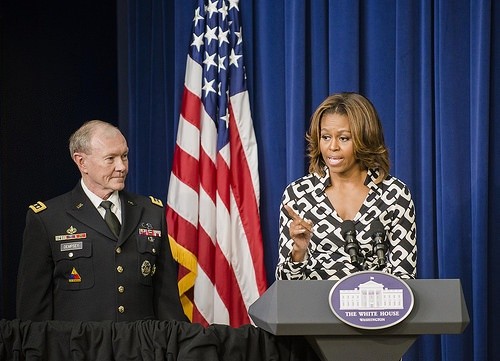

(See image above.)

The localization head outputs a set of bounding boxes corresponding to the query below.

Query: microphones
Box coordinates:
[340,220,358,265]
[370,221,387,267]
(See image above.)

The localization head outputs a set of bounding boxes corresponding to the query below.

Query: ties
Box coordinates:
[100,201,121,239]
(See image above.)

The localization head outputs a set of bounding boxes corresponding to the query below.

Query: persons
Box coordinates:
[275,94,417,281]
[17,121,189,322]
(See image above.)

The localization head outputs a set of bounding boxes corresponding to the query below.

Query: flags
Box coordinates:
[167,0,267,328]
